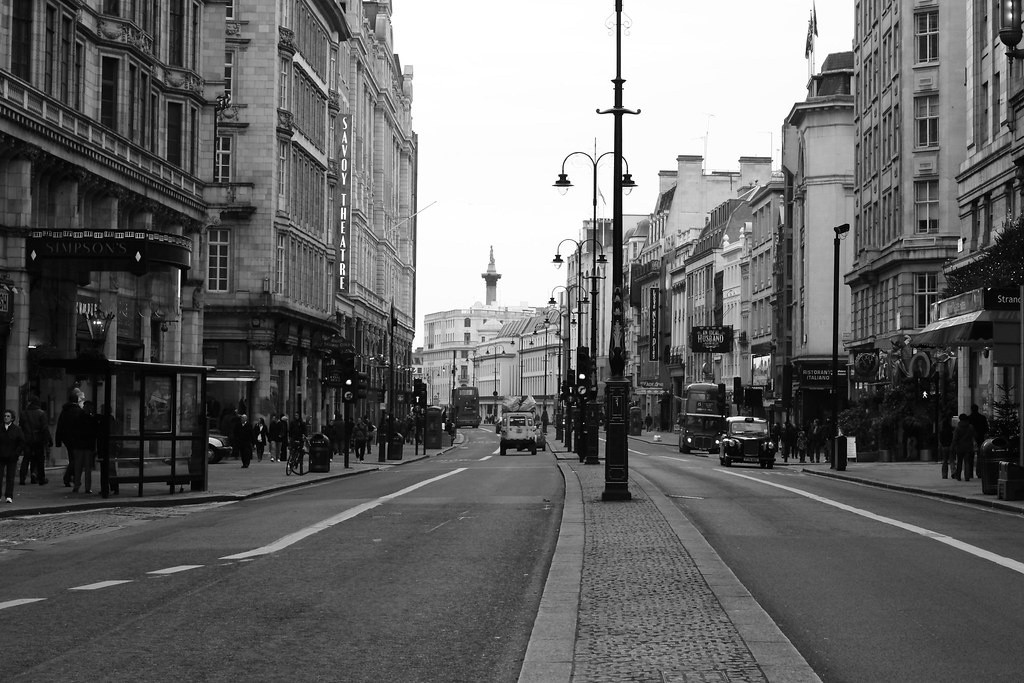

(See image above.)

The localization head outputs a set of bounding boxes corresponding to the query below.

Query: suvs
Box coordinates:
[500,412,537,455]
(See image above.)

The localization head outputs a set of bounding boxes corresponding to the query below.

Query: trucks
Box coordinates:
[501,396,537,412]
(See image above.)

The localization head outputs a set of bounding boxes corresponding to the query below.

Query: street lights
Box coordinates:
[466,355,484,386]
[830,223,850,470]
[443,361,458,404]
[551,136,638,465]
[486,342,505,416]
[544,309,577,440]
[426,368,440,406]
[511,333,534,396]
[549,286,590,453]
[533,316,559,434]
[552,232,609,460]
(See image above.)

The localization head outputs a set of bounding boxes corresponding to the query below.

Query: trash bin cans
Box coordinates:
[979,437,1013,494]
[309,434,331,472]
[835,436,847,471]
[387,433,404,460]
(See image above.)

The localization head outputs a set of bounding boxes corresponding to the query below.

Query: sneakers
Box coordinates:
[6,498,12,503]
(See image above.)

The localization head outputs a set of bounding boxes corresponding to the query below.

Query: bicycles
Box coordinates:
[286,434,314,475]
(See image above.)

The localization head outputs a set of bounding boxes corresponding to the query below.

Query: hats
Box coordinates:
[281,416,288,421]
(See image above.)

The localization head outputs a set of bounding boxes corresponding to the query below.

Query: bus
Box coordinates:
[452,387,482,428]
[678,383,726,454]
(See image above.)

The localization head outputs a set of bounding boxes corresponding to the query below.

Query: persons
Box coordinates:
[940,402,990,481]
[219,408,426,468]
[534,414,541,428]
[770,418,833,463]
[645,413,653,432]
[541,410,549,433]
[441,406,456,446]
[0,388,118,503]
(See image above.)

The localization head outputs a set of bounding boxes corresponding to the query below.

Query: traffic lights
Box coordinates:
[577,346,589,386]
[414,378,421,405]
[566,369,575,386]
[342,370,358,403]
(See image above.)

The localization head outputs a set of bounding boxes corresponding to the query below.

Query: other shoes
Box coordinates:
[271,457,274,462]
[39,479,49,486]
[277,460,280,463]
[62,477,71,487]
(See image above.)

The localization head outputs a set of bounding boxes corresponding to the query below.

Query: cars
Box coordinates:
[537,429,547,451]
[209,435,232,464]
[495,417,502,433]
[719,416,777,469]
